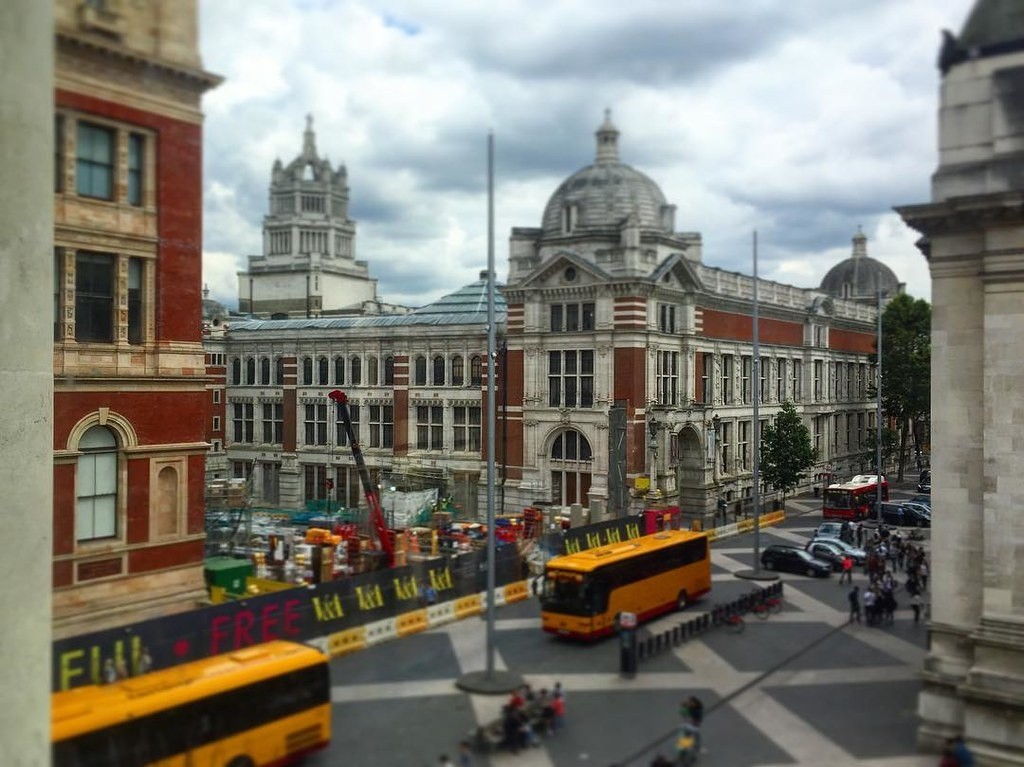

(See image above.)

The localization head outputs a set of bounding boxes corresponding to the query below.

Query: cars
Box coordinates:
[917,470,931,492]
[869,495,931,527]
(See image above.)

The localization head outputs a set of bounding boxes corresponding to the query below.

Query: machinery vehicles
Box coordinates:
[326,391,395,572]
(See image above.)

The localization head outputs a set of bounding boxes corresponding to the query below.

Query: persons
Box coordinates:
[680,696,703,728]
[840,521,862,548]
[503,682,565,752]
[861,524,928,595]
[917,458,922,471]
[909,589,922,624]
[735,501,741,516]
[849,571,897,626]
[718,496,725,516]
[839,555,853,584]
[440,741,472,767]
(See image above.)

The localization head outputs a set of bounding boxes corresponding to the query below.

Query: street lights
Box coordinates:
[648,416,665,509]
[713,414,725,502]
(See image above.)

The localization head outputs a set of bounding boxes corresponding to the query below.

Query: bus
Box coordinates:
[824,474,889,520]
[531,530,710,640]
[51,638,331,766]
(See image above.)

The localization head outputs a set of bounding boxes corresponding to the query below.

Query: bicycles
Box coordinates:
[737,588,782,619]
[712,601,745,634]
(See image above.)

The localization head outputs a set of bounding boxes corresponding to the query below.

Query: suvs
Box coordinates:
[761,522,900,578]
